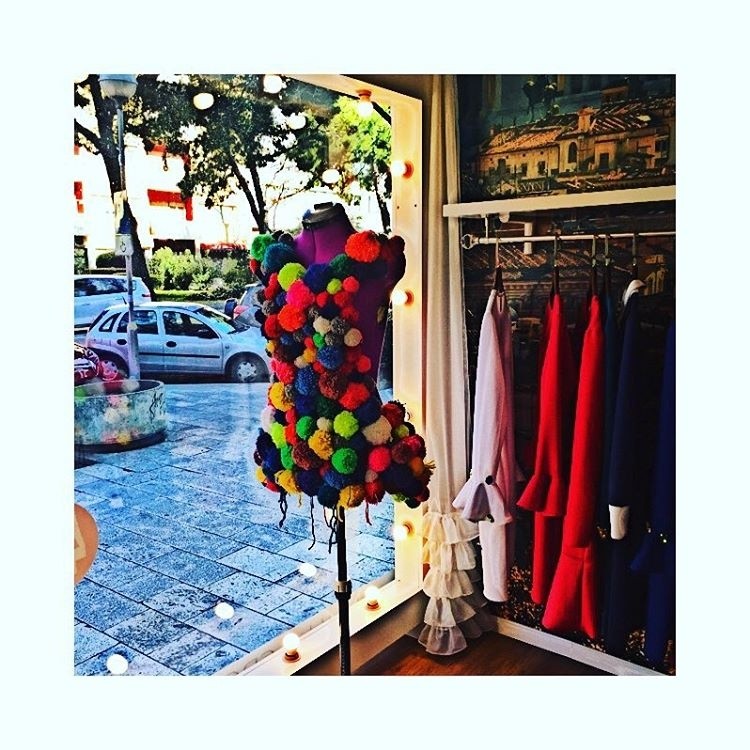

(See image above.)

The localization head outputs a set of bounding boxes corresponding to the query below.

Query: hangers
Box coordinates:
[482,231,675,322]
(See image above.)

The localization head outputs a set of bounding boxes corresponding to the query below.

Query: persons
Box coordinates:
[247,202,437,514]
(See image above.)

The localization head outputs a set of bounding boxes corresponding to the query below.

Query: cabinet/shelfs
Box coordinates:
[442,185,675,675]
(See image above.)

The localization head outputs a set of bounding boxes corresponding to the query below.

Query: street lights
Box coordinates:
[97,74,140,380]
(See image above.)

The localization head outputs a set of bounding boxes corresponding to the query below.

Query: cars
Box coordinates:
[73,342,101,386]
[84,300,274,385]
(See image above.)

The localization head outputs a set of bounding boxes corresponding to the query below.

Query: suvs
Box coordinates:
[223,283,267,329]
[74,273,151,328]
